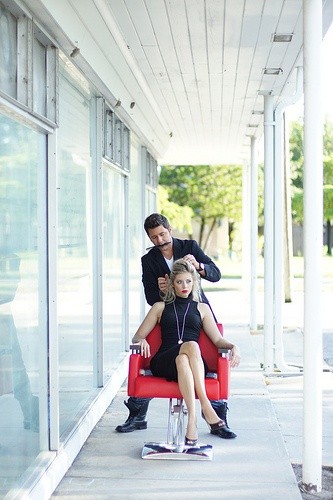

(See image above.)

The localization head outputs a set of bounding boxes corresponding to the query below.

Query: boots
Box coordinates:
[210,402,237,438]
[115,397,152,432]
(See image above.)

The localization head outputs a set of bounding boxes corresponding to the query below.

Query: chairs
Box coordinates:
[127,323,229,461]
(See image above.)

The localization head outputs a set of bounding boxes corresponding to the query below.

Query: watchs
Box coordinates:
[196,262,204,271]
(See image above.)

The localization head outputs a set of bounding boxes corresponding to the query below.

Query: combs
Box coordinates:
[146,242,171,251]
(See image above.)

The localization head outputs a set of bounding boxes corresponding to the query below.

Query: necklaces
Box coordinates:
[172,300,190,344]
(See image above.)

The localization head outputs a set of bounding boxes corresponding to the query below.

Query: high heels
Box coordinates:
[185,436,199,445]
[201,409,225,429]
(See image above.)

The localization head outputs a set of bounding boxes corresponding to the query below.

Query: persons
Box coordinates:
[115,213,237,433]
[132,258,241,446]
